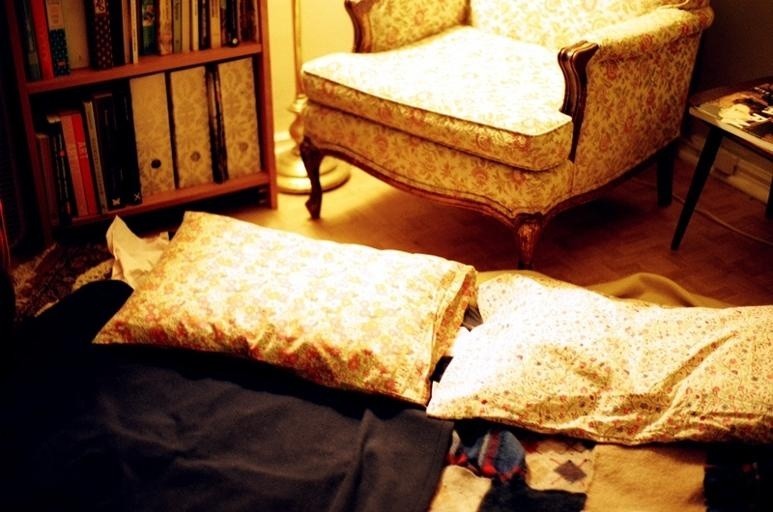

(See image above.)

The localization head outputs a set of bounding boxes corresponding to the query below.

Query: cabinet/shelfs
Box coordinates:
[0,0,277,248]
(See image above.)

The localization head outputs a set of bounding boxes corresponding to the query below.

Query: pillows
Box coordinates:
[89,211,475,407]
[438,271,773,449]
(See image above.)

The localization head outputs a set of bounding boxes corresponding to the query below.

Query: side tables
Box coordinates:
[670,79,773,252]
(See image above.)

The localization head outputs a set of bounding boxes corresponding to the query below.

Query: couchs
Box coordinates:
[299,0,718,270]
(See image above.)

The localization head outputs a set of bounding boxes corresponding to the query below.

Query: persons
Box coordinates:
[717,97,769,128]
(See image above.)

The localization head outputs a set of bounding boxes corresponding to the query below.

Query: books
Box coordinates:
[755,81,772,97]
[15,1,262,227]
[694,90,772,130]
